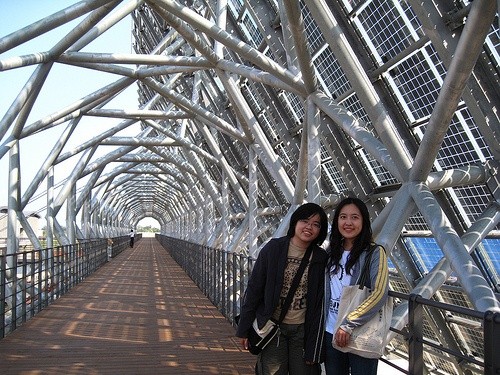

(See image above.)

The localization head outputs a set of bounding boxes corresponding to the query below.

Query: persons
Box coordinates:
[323,198,391,375]
[129,230,134,248]
[235,203,329,375]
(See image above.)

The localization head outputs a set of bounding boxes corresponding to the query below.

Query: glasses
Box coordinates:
[299,219,321,229]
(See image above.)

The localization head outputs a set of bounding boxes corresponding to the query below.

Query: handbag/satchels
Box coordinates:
[234,312,282,355]
[332,245,393,358]
